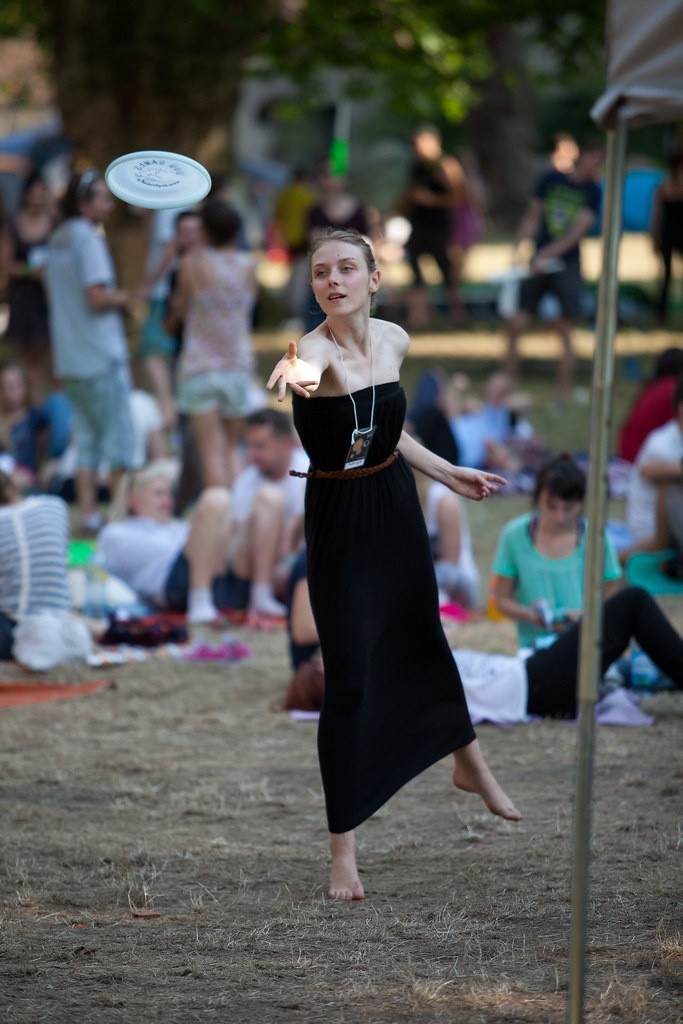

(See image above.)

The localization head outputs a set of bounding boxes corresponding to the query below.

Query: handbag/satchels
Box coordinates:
[12,614,89,670]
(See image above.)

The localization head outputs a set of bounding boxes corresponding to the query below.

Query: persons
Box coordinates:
[0,132,683,728]
[264,229,523,899]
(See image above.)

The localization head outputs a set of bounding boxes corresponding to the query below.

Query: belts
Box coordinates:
[288,450,397,479]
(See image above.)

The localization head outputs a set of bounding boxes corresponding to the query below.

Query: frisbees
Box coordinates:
[105,149,212,211]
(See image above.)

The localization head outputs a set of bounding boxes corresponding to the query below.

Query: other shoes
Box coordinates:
[99,614,166,647]
[81,517,106,536]
[152,614,186,646]
